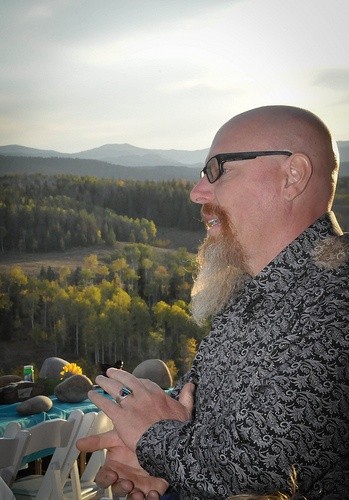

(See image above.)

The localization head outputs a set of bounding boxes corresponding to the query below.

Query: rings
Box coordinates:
[116,386,132,404]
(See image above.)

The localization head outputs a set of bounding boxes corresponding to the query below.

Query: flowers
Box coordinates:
[44,362,83,403]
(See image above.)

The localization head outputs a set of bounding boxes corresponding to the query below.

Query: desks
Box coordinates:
[48,388,172,476]
[0,402,61,475]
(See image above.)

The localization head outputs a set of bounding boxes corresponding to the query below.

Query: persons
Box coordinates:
[75,105,349,500]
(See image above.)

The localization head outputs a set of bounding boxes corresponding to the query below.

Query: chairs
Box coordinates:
[0,359,173,500]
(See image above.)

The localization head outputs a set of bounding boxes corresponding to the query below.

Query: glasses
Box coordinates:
[200,150,292,184]
